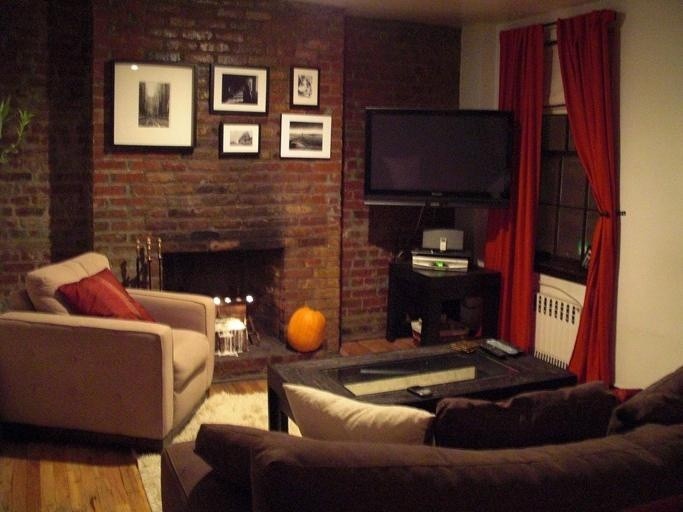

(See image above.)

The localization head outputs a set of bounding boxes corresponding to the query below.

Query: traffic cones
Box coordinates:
[286,306,327,352]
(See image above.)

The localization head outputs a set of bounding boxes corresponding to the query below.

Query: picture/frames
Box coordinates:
[385,257,502,347]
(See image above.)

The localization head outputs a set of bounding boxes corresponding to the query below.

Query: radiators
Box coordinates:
[480,343,506,357]
[487,340,518,354]
[498,340,524,353]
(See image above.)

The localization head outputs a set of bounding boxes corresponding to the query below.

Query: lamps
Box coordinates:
[0,251,216,454]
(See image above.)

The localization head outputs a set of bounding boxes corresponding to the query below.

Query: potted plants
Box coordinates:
[280,113,332,159]
[220,121,261,157]
[290,64,320,109]
[108,57,195,154]
[209,63,269,115]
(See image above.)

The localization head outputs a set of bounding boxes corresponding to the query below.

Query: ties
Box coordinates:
[364,108,514,209]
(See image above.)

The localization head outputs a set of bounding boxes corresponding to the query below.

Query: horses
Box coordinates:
[407,386,433,397]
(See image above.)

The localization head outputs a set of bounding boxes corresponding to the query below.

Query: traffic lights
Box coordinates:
[194,423,268,492]
[282,381,435,446]
[433,377,618,450]
[58,267,155,322]
[605,365,682,437]
[26,251,110,315]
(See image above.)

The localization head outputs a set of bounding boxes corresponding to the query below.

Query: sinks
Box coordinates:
[159,365,682,512]
